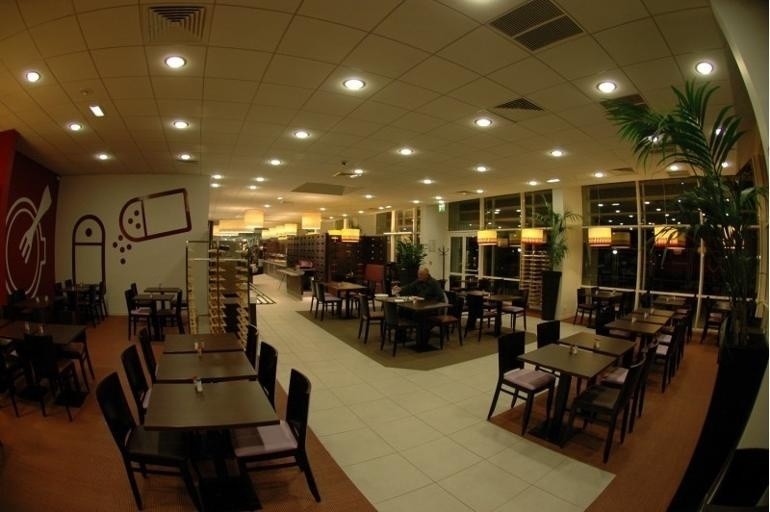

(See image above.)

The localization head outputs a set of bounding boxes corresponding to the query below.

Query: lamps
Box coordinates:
[588,190,611,246]
[521,191,547,243]
[655,184,686,248]
[477,196,496,245]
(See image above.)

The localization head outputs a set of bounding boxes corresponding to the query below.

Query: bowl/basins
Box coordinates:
[374,294,389,301]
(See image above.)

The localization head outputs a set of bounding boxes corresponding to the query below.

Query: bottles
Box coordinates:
[593,337,601,350]
[193,376,204,394]
[569,344,577,356]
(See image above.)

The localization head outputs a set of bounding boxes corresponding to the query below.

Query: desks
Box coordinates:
[277,269,305,297]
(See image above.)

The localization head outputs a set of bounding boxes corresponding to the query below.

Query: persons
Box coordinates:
[393,267,446,347]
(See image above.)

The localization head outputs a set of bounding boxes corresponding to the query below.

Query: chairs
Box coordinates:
[0,278,109,421]
[139,328,158,384]
[228,369,321,505]
[700,296,722,345]
[95,371,204,511]
[536,321,561,379]
[245,324,258,369]
[310,276,528,357]
[124,283,187,341]
[121,343,148,426]
[487,330,556,435]
[255,342,277,407]
[560,287,694,464]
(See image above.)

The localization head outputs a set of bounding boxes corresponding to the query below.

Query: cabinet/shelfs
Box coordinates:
[289,234,386,293]
[186,240,251,351]
[520,251,550,310]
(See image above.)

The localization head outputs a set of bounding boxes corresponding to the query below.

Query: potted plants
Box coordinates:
[397,236,428,283]
[534,193,584,322]
